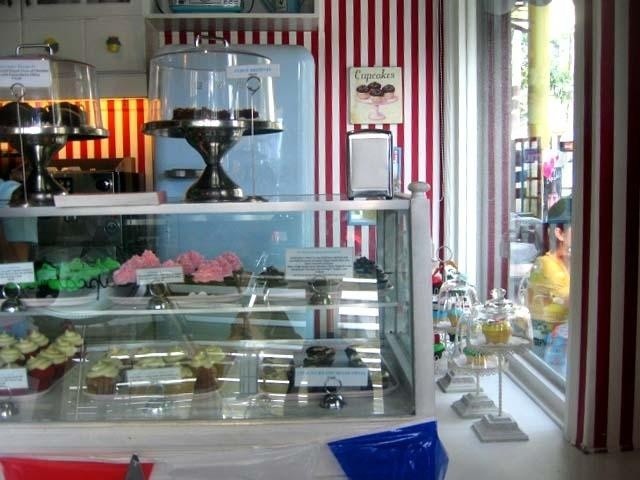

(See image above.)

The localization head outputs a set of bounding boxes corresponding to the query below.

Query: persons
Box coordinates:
[526,195,577,371]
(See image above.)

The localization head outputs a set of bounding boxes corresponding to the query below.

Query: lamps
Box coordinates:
[106,35,122,54]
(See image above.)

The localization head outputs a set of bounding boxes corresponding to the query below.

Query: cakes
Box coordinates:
[0,249,243,393]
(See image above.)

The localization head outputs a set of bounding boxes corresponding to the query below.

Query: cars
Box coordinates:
[510,242,537,299]
[509,216,546,249]
